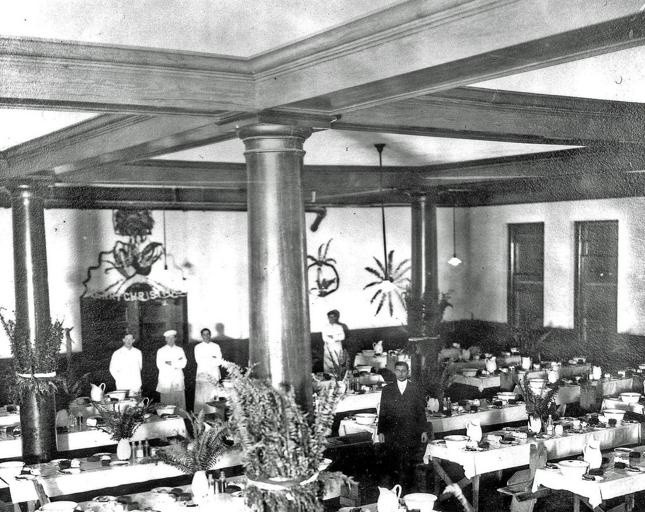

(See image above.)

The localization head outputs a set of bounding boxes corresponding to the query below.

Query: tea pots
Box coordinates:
[90,382,105,401]
[377,484,402,512]
[372,340,383,355]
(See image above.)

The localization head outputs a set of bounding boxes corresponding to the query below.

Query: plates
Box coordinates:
[319,350,645,480]
[0,400,374,511]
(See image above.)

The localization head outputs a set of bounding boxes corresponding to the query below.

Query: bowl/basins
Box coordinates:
[404,492,438,512]
[107,389,128,400]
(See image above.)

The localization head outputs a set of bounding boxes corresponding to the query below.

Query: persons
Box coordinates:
[380,362,427,493]
[109,334,143,401]
[155,329,187,410]
[194,328,223,414]
[322,311,345,372]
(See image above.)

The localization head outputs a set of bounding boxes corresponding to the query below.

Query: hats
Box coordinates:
[162,329,178,337]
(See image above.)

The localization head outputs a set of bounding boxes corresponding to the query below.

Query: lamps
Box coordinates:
[447,204,462,267]
[364,143,411,316]
